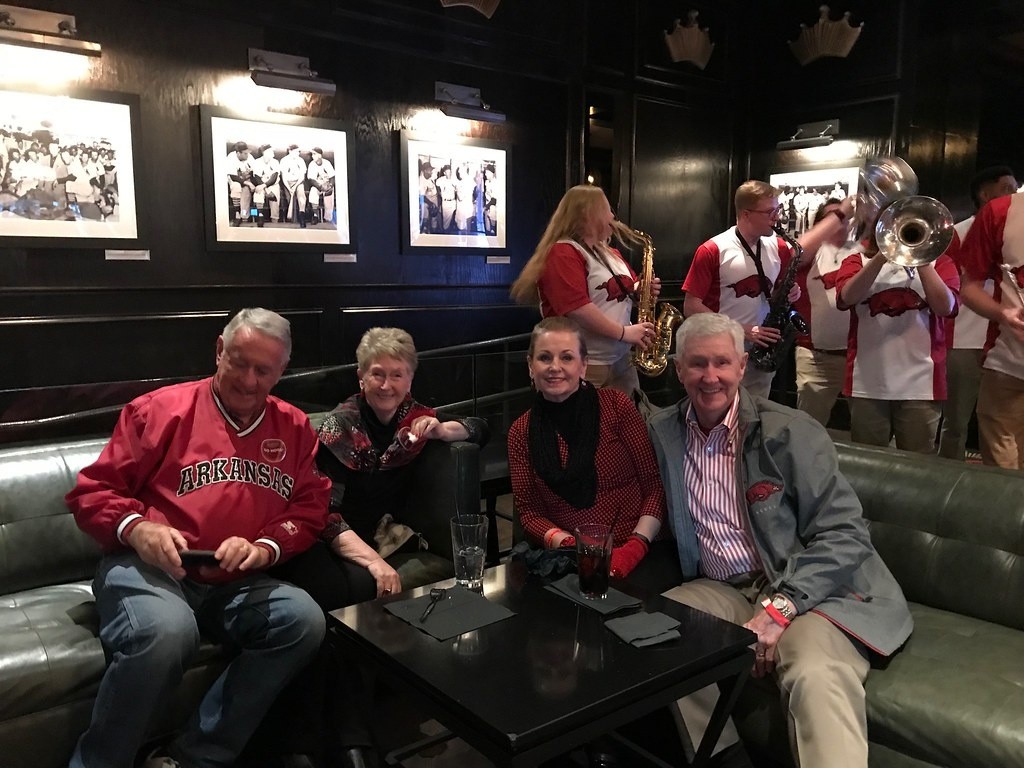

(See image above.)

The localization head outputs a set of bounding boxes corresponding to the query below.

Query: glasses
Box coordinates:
[747,208,780,218]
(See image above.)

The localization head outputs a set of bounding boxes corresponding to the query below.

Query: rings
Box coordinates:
[756,653,765,658]
[385,585,391,591]
[243,544,249,552]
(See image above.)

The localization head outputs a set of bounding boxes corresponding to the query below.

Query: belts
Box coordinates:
[445,199,455,202]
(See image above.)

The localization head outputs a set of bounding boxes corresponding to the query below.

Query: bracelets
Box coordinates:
[618,325,625,342]
[760,597,791,630]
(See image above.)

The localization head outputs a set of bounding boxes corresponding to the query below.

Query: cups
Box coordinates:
[450,513,489,595]
[576,523,614,600]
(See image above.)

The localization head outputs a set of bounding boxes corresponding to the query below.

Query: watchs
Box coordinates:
[772,596,796,621]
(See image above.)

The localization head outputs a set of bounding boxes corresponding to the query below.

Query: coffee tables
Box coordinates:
[330,559,758,768]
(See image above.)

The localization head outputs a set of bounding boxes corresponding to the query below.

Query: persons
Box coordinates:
[958,192,1024,470]
[226,141,336,228]
[645,312,914,768]
[507,316,667,582]
[510,186,661,399]
[681,180,802,400]
[836,200,961,455]
[315,326,491,598]
[527,636,580,704]
[936,165,1019,460]
[64,309,332,768]
[418,159,497,236]
[0,129,118,222]
[786,196,871,428]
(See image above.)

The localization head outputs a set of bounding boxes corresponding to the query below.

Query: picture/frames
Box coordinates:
[0,78,152,252]
[398,129,515,256]
[199,102,360,256]
[762,157,867,243]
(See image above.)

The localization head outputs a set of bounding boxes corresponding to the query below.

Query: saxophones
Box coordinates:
[748,225,809,373]
[609,219,685,376]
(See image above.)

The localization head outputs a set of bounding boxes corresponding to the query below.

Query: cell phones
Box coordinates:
[180,549,221,567]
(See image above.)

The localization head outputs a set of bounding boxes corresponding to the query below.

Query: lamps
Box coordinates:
[248,47,337,98]
[776,118,841,151]
[435,81,507,123]
[0,5,104,60]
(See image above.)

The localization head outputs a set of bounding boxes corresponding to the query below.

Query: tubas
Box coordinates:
[860,155,955,267]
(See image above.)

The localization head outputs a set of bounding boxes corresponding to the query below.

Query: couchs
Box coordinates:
[830,438,1024,768]
[0,412,482,768]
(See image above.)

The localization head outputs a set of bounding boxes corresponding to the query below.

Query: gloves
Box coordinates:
[561,535,648,579]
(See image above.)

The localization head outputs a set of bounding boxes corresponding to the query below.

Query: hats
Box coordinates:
[484,165,495,173]
[423,162,435,170]
[236,142,322,155]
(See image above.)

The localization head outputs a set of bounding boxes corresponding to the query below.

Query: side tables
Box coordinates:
[476,432,513,571]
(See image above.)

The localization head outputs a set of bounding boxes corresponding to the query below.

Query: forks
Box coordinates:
[420,588,445,623]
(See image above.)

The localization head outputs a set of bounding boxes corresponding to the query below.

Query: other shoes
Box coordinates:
[235,212,318,228]
